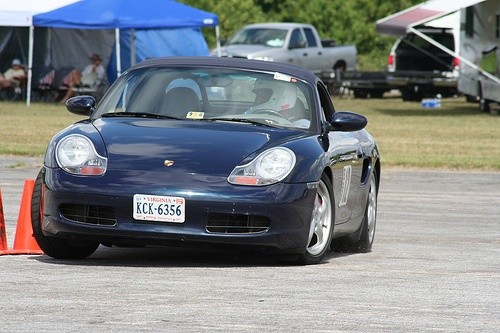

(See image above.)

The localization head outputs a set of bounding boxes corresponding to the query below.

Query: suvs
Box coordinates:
[385,23,461,101]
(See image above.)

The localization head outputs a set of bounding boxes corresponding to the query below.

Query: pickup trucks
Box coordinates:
[209,20,358,96]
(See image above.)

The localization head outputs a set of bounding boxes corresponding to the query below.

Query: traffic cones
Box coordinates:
[0,181,9,255]
[8,177,47,256]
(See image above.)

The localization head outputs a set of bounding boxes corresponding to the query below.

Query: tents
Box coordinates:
[0,0,221,111]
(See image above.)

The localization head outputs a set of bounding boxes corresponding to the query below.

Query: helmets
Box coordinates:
[250,76,297,111]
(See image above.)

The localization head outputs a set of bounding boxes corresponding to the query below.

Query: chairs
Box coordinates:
[321,71,362,96]
[156,73,210,117]
[0,66,107,103]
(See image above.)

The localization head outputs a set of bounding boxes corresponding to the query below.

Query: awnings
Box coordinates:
[376,0,500,83]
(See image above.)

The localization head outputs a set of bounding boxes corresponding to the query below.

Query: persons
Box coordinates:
[0,59,26,89]
[251,79,305,122]
[61,54,105,103]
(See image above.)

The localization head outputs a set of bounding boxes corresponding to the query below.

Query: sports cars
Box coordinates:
[29,52,382,266]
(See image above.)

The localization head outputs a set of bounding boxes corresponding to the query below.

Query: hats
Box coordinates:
[13,59,20,65]
[88,54,102,60]
